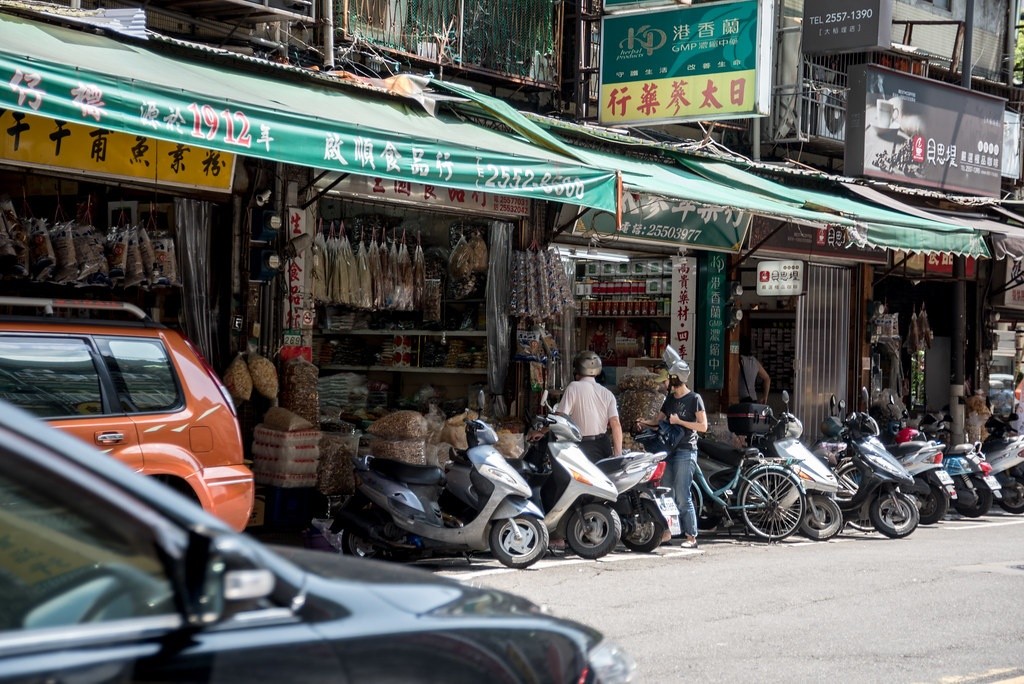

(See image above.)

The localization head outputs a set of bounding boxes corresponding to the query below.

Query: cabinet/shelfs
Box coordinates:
[313,294,510,431]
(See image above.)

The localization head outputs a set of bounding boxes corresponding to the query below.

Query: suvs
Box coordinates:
[0,296,254,534]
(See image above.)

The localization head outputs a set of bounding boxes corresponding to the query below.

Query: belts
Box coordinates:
[579,432,609,443]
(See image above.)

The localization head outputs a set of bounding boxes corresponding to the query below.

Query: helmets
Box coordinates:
[572,350,602,376]
[895,426,927,445]
[668,360,691,383]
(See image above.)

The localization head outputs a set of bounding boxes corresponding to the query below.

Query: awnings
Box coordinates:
[662,152,991,259]
[867,199,1024,263]
[0,11,622,231]
[501,131,868,249]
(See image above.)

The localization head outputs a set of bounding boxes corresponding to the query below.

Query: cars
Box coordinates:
[0,402,640,683]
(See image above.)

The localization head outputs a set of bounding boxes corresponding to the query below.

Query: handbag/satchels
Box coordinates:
[632,417,686,459]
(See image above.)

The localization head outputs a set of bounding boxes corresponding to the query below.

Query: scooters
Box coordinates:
[328,390,549,568]
[685,385,1024,542]
[516,407,680,553]
[439,391,623,561]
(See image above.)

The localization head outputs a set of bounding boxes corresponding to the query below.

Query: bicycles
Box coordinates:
[672,446,807,544]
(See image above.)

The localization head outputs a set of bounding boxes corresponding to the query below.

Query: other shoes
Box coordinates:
[681,539,698,549]
[660,528,672,547]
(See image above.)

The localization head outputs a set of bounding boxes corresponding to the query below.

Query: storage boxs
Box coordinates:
[247,490,267,527]
[800,84,846,142]
[726,403,771,434]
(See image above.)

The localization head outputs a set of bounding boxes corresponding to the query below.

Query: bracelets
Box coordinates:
[540,431,545,434]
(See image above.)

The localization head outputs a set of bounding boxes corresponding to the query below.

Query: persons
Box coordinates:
[529,350,623,465]
[635,343,708,548]
[738,336,772,405]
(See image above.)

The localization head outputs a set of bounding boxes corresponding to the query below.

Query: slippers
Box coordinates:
[544,548,565,553]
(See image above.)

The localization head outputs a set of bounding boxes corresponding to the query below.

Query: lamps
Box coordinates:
[671,246,688,268]
[587,234,600,255]
